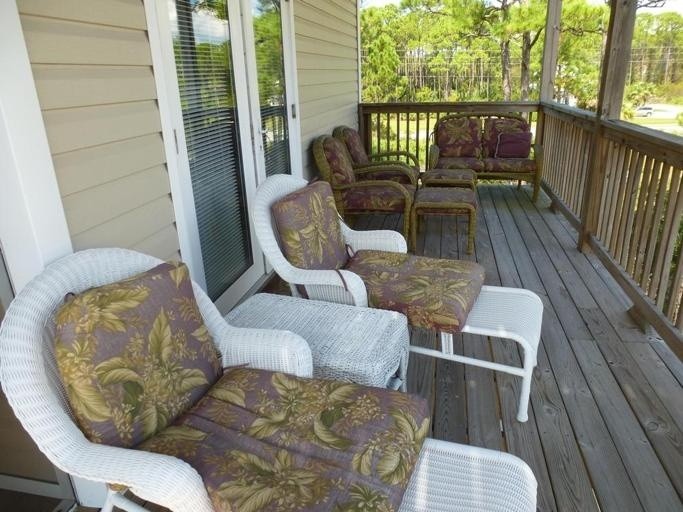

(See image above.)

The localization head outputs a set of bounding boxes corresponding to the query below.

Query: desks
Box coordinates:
[223,292,409,394]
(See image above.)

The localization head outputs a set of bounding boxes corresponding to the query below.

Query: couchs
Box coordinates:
[429,112,544,202]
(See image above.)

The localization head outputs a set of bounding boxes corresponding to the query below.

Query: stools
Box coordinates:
[411,187,478,254]
[422,168,477,194]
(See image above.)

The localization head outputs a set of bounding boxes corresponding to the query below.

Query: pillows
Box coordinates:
[55,258,225,447]
[271,180,349,271]
[495,131,532,160]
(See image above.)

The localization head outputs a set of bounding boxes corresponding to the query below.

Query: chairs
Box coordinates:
[0,247,538,512]
[252,173,544,422]
[333,126,420,185]
[313,134,418,243]
